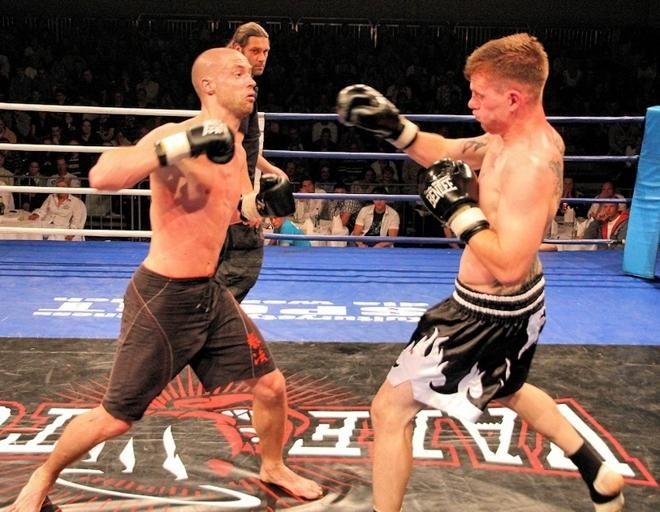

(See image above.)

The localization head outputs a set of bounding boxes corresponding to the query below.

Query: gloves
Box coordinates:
[237,174,296,222]
[154,120,235,167]
[419,158,490,245]
[338,84,419,151]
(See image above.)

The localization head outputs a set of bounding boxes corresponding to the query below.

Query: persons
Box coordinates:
[0,48,322,512]
[213,22,290,303]
[335,34,624,512]
[0,47,660,248]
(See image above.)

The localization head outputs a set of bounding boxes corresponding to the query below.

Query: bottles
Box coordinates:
[0,196,5,214]
[314,208,320,228]
